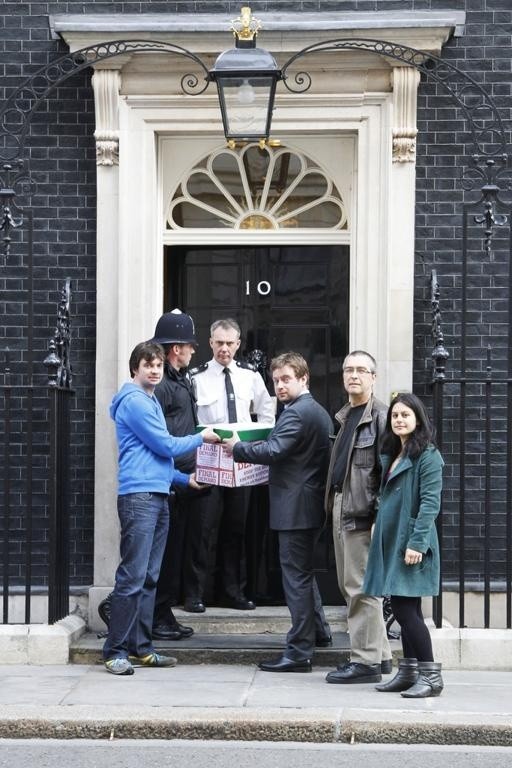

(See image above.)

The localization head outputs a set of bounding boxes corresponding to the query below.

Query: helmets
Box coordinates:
[152,308,196,343]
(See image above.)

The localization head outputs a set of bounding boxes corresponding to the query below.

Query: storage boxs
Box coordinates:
[194,422,276,488]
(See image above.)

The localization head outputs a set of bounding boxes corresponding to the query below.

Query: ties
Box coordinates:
[223,368,237,423]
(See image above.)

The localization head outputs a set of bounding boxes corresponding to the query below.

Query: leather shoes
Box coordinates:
[106,597,204,674]
[259,635,443,697]
[232,600,255,609]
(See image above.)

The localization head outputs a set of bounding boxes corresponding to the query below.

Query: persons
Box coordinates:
[324,350,395,684]
[97,306,200,641]
[361,393,445,698]
[179,318,276,613]
[224,351,335,673]
[102,340,222,675]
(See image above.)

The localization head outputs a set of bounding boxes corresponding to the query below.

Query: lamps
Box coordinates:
[201,6,292,157]
